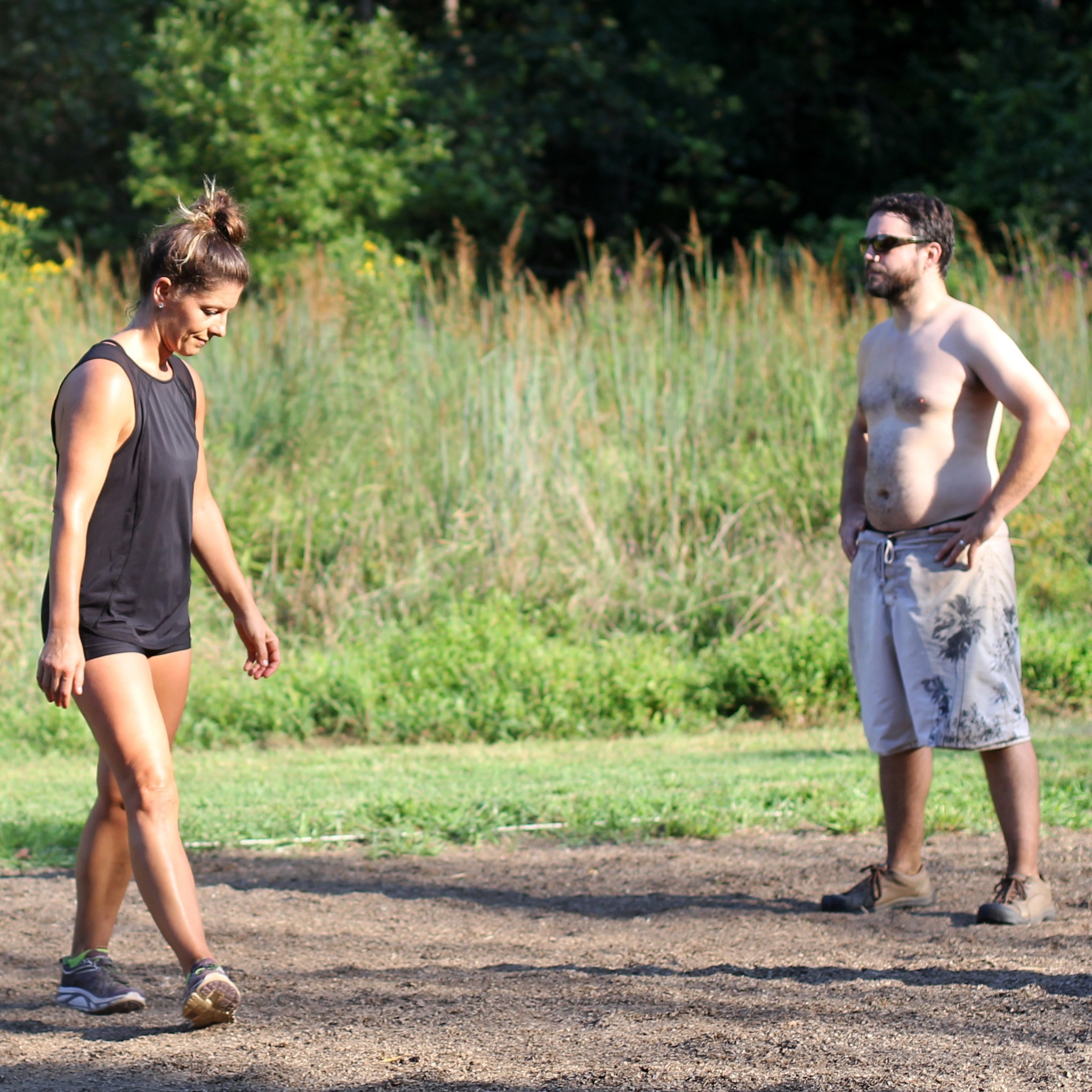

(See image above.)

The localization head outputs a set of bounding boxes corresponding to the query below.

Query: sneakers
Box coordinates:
[820,863,934,914]
[55,948,147,1015]
[180,956,241,1024]
[976,872,1057,925]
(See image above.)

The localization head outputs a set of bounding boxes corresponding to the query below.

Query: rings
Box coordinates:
[959,540,967,546]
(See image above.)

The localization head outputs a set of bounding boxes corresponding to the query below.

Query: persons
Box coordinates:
[36,175,282,1025]
[820,193,1072,929]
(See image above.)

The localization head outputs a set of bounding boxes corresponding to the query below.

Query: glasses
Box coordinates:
[858,234,932,257]
